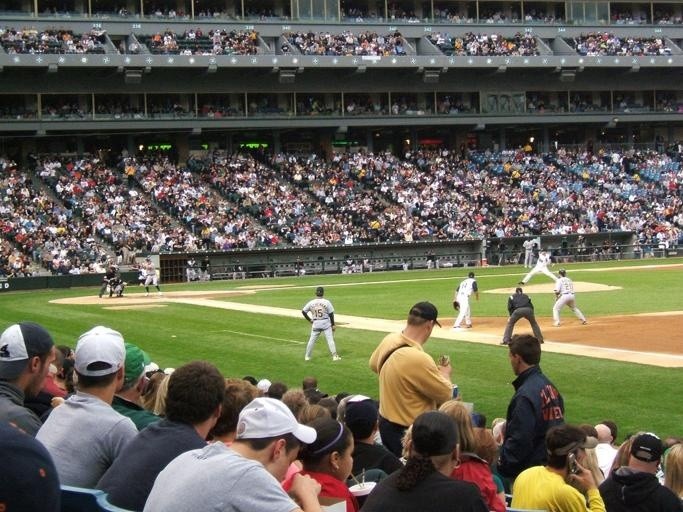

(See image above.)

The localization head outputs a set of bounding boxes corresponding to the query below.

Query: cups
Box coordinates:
[347,481,378,510]
[438,353,450,368]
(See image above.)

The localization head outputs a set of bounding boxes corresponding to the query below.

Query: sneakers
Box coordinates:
[118,342,152,383]
[536,338,545,344]
[333,355,342,361]
[304,356,311,361]
[500,339,512,346]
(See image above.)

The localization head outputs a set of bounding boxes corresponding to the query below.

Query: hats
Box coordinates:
[235,395,318,445]
[630,433,662,463]
[342,394,378,441]
[546,424,599,457]
[558,269,567,276]
[146,361,350,407]
[315,287,324,296]
[408,301,443,328]
[1,321,56,381]
[73,325,127,378]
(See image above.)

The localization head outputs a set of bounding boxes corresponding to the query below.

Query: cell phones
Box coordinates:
[569,453,581,475]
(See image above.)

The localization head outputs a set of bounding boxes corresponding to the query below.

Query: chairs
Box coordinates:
[1,32,673,56]
